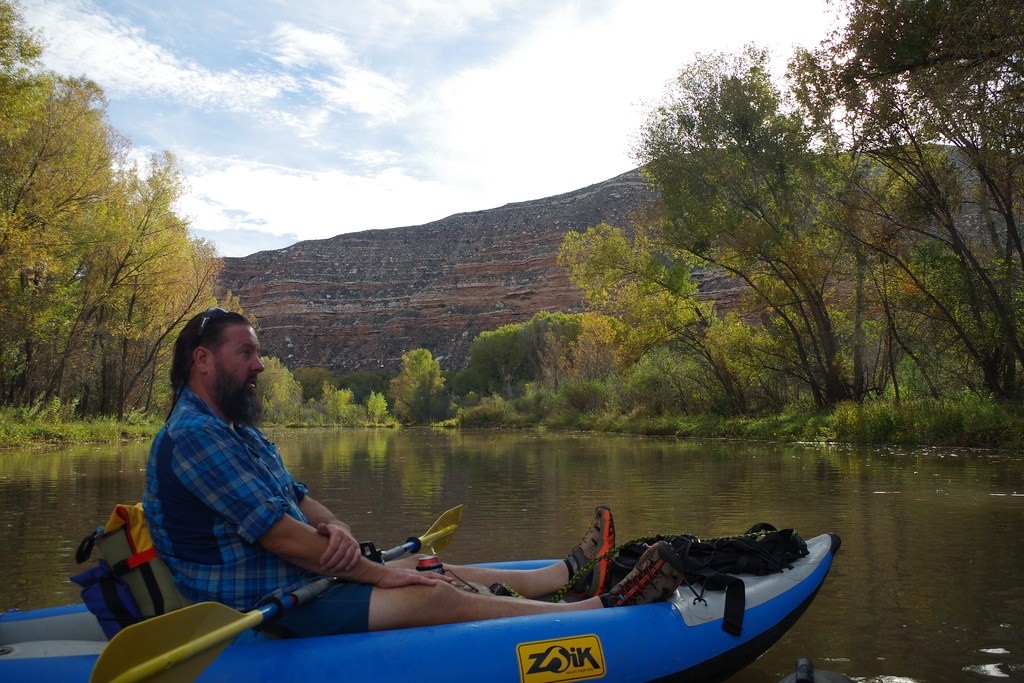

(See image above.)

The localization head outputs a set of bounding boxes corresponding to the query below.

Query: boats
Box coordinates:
[0,529,845,683]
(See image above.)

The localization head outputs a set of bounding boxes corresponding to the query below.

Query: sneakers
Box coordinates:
[568,506,615,599]
[609,542,684,606]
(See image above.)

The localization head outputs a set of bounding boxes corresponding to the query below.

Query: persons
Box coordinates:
[136,313,689,640]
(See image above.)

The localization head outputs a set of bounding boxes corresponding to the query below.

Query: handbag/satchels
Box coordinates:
[75,503,188,640]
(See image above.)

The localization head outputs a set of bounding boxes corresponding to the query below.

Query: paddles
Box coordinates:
[88,503,466,682]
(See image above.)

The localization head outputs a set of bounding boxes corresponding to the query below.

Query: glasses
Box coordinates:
[198,307,228,336]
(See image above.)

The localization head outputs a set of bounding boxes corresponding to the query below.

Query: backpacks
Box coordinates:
[604,522,810,637]
